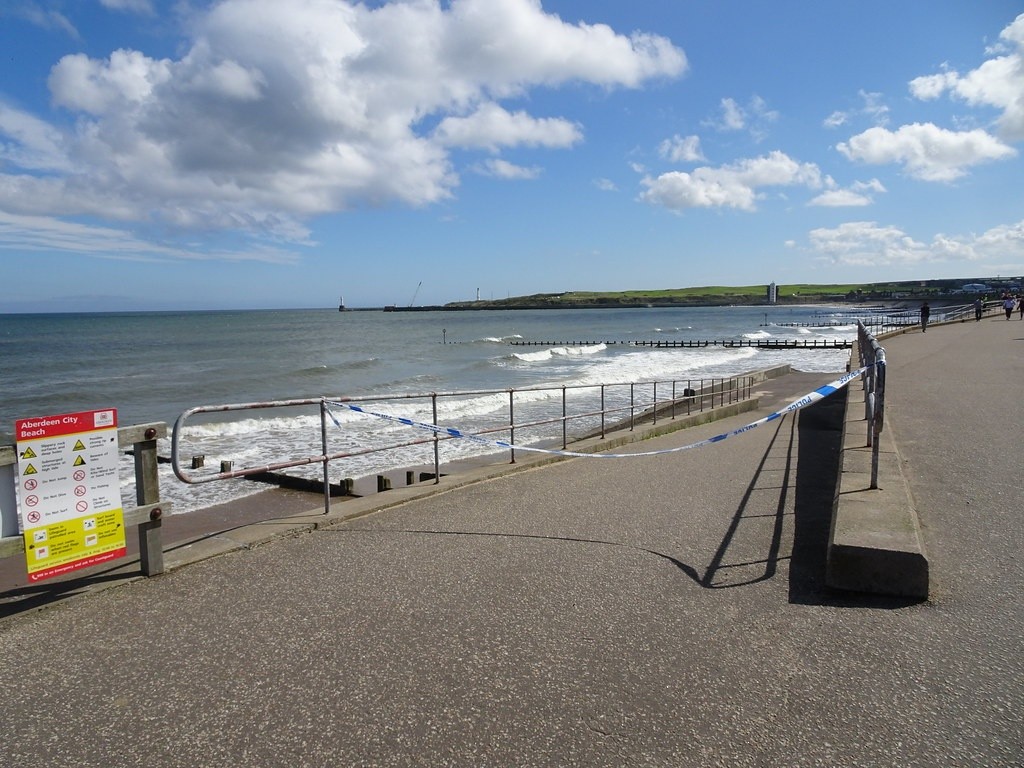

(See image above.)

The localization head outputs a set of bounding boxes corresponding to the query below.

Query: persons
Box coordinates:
[1003,296,1016,321]
[921,303,930,333]
[982,293,989,312]
[1001,292,1021,309]
[974,296,983,322]
[1017,294,1024,320]
